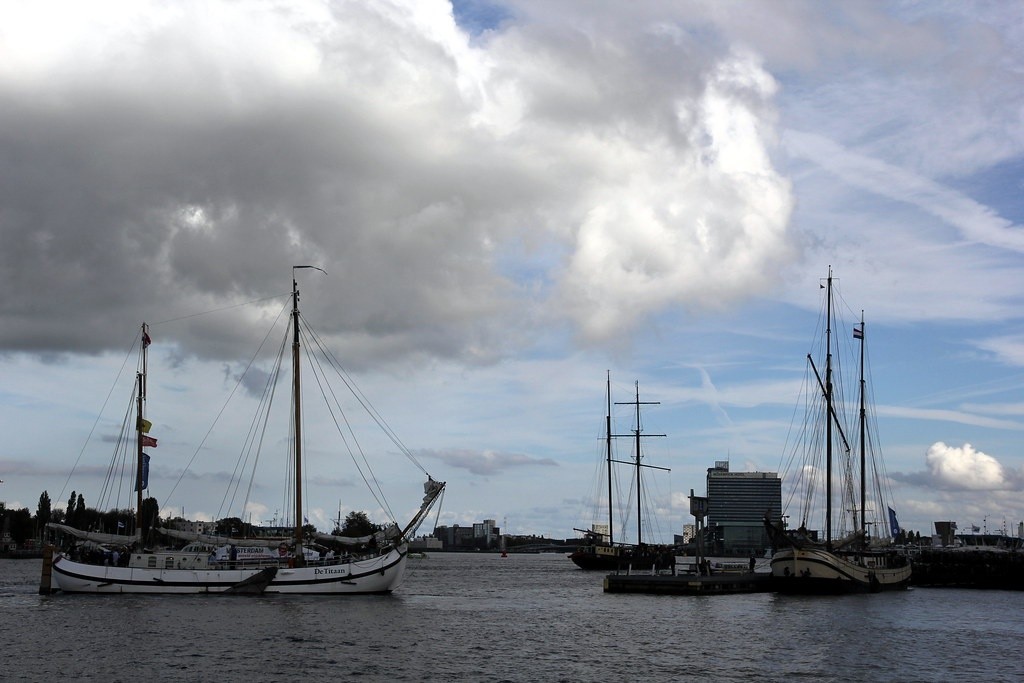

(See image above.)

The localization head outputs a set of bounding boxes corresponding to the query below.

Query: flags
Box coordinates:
[888,508,900,538]
[950,523,958,530]
[134,452,150,491]
[820,284,825,288]
[293,265,328,275]
[852,327,863,339]
[136,416,152,433]
[143,332,152,350]
[972,525,980,533]
[141,434,157,447]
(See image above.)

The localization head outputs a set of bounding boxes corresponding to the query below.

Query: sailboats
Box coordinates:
[44,264,447,596]
[567,370,674,571]
[762,264,911,594]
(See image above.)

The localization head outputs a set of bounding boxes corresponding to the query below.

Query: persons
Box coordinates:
[108,546,130,567]
[750,548,756,556]
[699,559,713,576]
[210,551,220,564]
[70,542,78,561]
[230,544,237,570]
[368,535,377,558]
[180,539,188,550]
[750,554,757,573]
[341,546,349,563]
[326,549,334,565]
[80,548,106,566]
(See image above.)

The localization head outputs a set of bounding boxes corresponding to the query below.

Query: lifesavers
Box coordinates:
[278,542,289,555]
